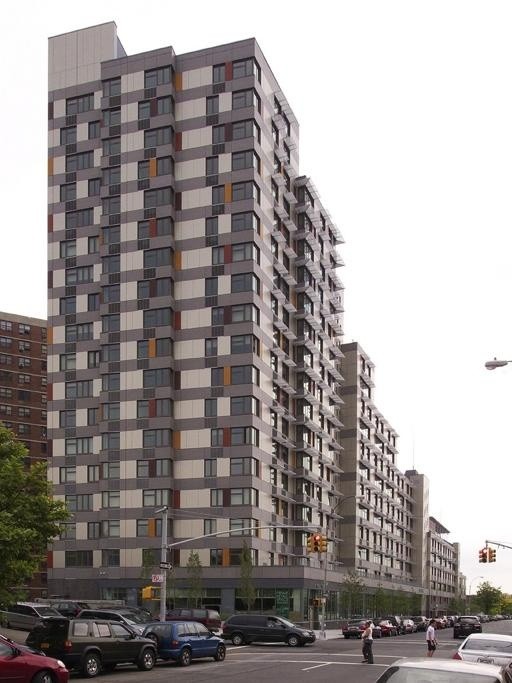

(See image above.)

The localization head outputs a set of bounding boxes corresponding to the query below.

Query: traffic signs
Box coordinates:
[160,561,174,571]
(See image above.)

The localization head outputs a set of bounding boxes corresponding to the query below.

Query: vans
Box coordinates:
[221,613,316,646]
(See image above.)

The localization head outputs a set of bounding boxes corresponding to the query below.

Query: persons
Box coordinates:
[425,619,438,657]
[360,621,374,663]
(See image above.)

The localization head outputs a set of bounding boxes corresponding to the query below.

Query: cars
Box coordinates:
[373,655,506,682]
[451,631,512,682]
[0,596,226,683]
[343,613,511,639]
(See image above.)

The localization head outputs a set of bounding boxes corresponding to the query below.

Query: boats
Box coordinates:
[313,534,322,552]
[307,535,314,551]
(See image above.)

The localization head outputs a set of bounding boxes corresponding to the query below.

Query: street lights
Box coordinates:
[468,575,483,614]
[330,495,364,517]
[485,355,511,369]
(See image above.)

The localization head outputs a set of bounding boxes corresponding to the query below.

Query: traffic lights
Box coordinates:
[312,597,320,607]
[320,535,327,552]
[481,551,487,563]
[488,549,495,562]
[479,548,484,563]
